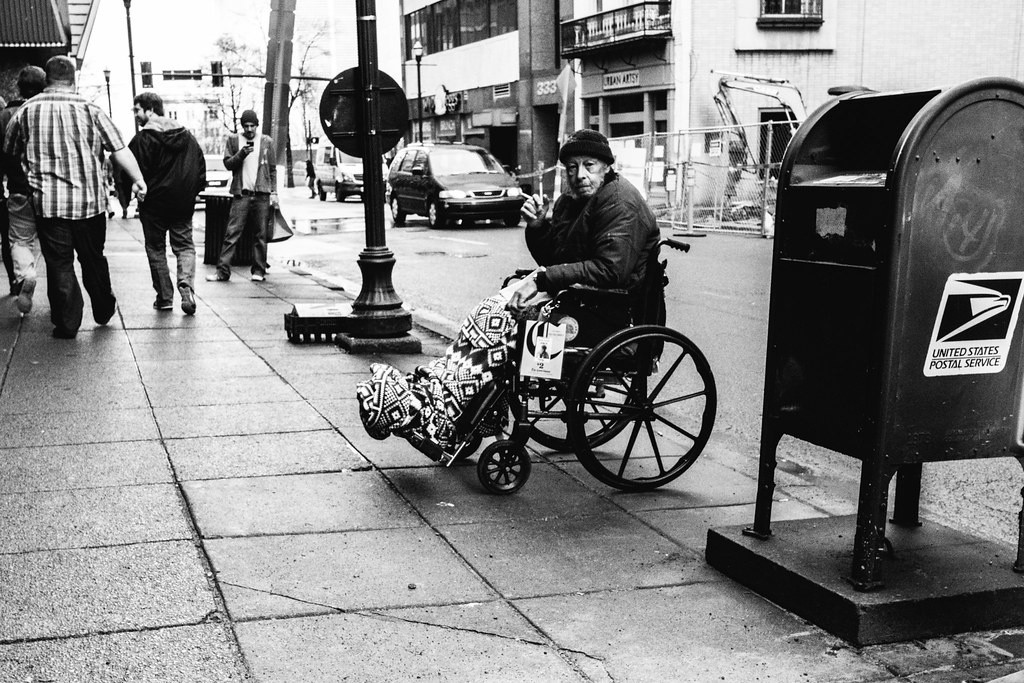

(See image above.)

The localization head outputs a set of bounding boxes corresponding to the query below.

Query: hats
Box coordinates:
[559,128,615,166]
[241,110,259,126]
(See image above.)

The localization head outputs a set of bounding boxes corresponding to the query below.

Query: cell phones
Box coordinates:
[247,141,254,147]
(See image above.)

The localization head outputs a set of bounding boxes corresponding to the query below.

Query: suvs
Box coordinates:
[385,140,527,230]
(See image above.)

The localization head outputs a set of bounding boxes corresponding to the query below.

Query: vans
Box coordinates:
[316,134,390,203]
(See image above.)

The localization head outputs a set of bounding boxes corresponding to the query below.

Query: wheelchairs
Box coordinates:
[406,237,722,497]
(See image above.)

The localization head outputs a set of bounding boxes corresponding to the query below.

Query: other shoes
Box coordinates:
[178,283,197,315]
[10,280,23,295]
[251,270,264,281]
[17,278,37,313]
[93,295,116,325]
[205,273,229,281]
[122,209,127,219]
[52,327,77,340]
[152,300,174,310]
[109,212,114,219]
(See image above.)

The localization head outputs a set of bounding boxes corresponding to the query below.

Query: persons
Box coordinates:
[0,56,147,338]
[207,109,278,283]
[303,158,318,199]
[355,127,661,465]
[110,151,134,219]
[120,92,206,315]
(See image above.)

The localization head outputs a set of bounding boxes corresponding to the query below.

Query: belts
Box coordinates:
[241,190,270,197]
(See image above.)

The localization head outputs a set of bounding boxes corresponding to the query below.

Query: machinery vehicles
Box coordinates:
[712,73,809,211]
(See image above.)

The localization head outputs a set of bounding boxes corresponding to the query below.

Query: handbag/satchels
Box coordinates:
[266,205,294,244]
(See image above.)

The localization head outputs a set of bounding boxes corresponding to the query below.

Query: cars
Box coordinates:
[194,154,234,210]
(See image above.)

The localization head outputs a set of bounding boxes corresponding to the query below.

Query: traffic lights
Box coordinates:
[311,137,320,145]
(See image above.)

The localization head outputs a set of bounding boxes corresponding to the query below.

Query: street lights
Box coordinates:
[412,42,427,141]
[103,69,113,119]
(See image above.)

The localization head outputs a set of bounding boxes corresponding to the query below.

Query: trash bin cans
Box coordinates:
[742,72,1023,595]
[200,194,268,267]
